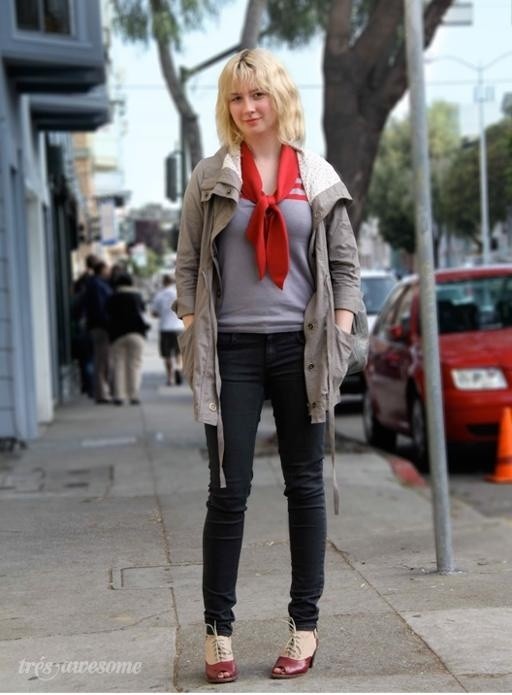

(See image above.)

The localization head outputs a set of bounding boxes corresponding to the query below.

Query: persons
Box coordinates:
[74,254,185,405]
[171,46,370,683]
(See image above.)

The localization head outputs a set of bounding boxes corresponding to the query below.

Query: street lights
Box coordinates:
[425,50,512,262]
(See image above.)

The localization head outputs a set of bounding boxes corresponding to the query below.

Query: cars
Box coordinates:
[339,263,512,465]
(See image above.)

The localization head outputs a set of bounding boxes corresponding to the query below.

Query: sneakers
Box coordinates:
[269,629,321,681]
[174,367,184,385]
[204,631,238,684]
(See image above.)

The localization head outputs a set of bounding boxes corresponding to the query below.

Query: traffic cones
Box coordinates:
[485,407,512,484]
